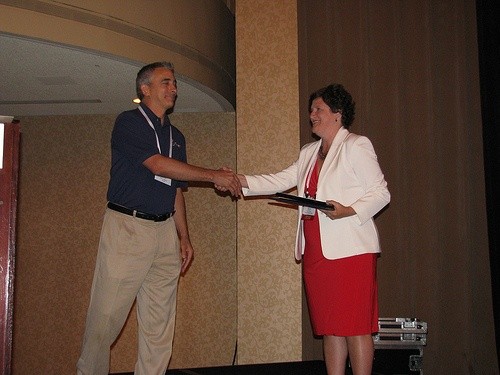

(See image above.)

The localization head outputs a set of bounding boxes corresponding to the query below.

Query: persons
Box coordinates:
[214,83,392,374]
[75,61,242,375]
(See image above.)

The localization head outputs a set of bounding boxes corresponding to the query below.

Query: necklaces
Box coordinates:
[317,146,330,159]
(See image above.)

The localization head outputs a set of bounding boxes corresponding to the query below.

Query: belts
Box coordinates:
[107,202,177,222]
